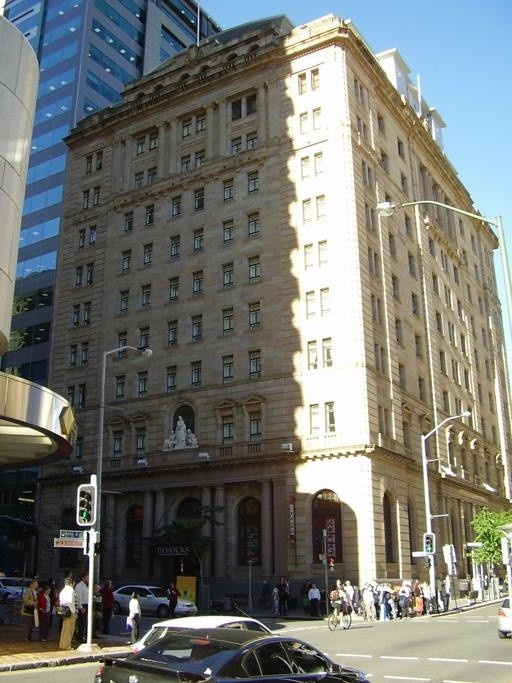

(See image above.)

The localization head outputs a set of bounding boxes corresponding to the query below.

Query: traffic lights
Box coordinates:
[327,556,336,571]
[423,532,435,553]
[76,485,97,526]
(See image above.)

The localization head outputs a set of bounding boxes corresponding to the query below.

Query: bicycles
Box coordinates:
[326,593,351,629]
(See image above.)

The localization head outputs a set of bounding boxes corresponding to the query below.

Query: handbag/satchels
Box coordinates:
[57,605,70,616]
[23,604,34,613]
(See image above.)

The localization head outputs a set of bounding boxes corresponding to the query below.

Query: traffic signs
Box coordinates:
[59,529,87,539]
[54,537,86,549]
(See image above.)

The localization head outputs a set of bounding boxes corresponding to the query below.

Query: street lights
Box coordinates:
[420,409,473,612]
[245,559,255,614]
[375,199,512,342]
[86,344,153,644]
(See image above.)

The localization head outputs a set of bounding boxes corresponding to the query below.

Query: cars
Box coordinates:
[1,576,31,605]
[497,597,511,638]
[108,584,199,618]
[134,616,273,658]
[91,628,372,683]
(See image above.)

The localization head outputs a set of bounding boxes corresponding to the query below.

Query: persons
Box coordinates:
[175,415,187,443]
[271,576,451,627]
[167,581,181,617]
[19,570,116,650]
[128,591,141,643]
[186,429,195,443]
[168,430,175,442]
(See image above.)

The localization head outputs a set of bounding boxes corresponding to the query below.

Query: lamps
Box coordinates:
[197,451,213,462]
[72,465,83,473]
[137,458,149,467]
[280,442,295,452]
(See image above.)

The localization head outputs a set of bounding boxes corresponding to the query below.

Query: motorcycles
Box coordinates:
[209,594,241,616]
